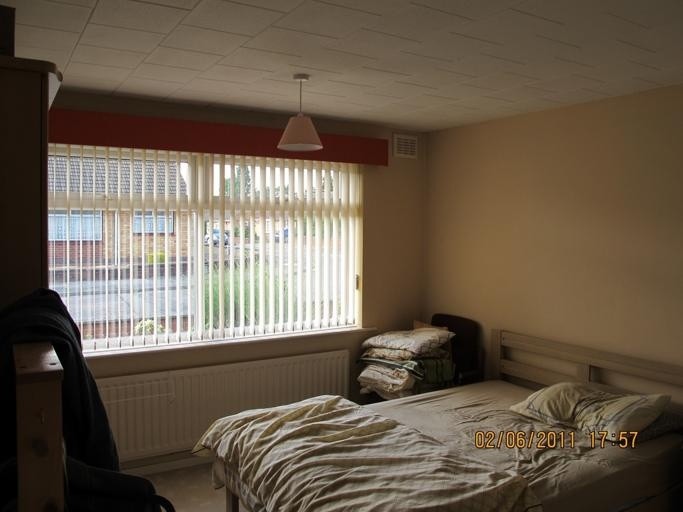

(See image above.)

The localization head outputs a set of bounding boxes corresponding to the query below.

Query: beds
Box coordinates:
[188,328,683,512]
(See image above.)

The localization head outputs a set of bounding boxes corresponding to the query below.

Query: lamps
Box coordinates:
[276,74,324,152]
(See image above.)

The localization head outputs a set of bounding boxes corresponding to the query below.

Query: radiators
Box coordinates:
[90,350,351,463]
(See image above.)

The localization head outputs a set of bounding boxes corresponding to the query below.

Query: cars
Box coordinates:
[204,229,228,246]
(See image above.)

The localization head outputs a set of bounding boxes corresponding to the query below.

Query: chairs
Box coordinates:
[429,312,485,392]
